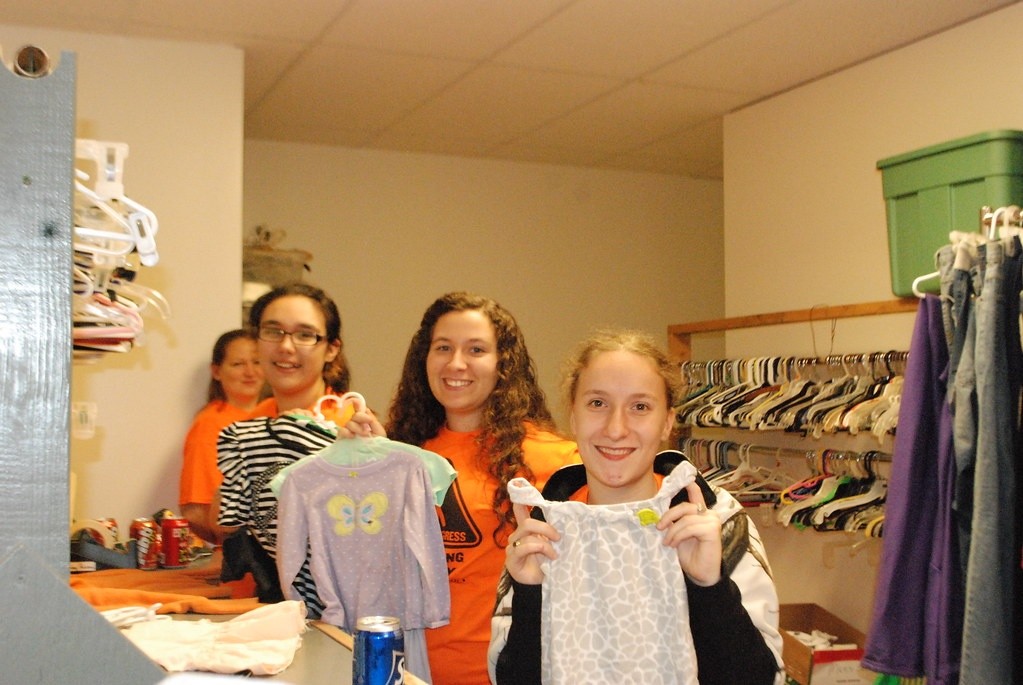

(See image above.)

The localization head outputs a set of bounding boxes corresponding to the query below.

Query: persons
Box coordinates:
[206,282,351,599]
[338,292,583,685]
[178,329,267,546]
[487,330,786,685]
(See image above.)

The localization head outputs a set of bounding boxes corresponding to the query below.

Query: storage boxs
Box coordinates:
[778,602,872,685]
[876,129,1023,299]
[242,245,314,314]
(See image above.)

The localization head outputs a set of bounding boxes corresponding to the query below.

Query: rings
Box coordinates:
[513,538,521,548]
[696,503,707,517]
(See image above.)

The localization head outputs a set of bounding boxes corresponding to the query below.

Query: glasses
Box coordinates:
[255,325,329,345]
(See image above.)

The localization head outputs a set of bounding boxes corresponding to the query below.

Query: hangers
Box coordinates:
[672,348,913,446]
[683,438,886,537]
[912,207,1010,299]
[314,392,384,464]
[74,137,171,364]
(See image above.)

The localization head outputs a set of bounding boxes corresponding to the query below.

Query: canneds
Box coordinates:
[98,518,188,569]
[353,616,405,685]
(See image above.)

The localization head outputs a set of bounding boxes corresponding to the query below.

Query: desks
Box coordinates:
[117,551,433,685]
